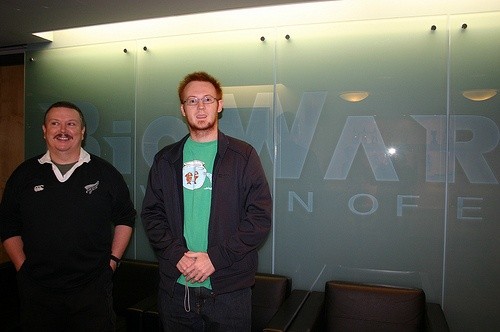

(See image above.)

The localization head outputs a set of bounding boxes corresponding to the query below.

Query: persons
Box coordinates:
[142,72,272,331]
[0,100,137,332]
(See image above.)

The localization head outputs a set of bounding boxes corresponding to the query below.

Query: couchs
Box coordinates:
[284,280,452,332]
[247,271,311,332]
[110,258,168,332]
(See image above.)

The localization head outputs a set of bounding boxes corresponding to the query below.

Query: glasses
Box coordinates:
[183,96,219,105]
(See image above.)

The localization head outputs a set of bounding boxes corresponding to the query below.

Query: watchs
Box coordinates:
[110,254,122,267]
